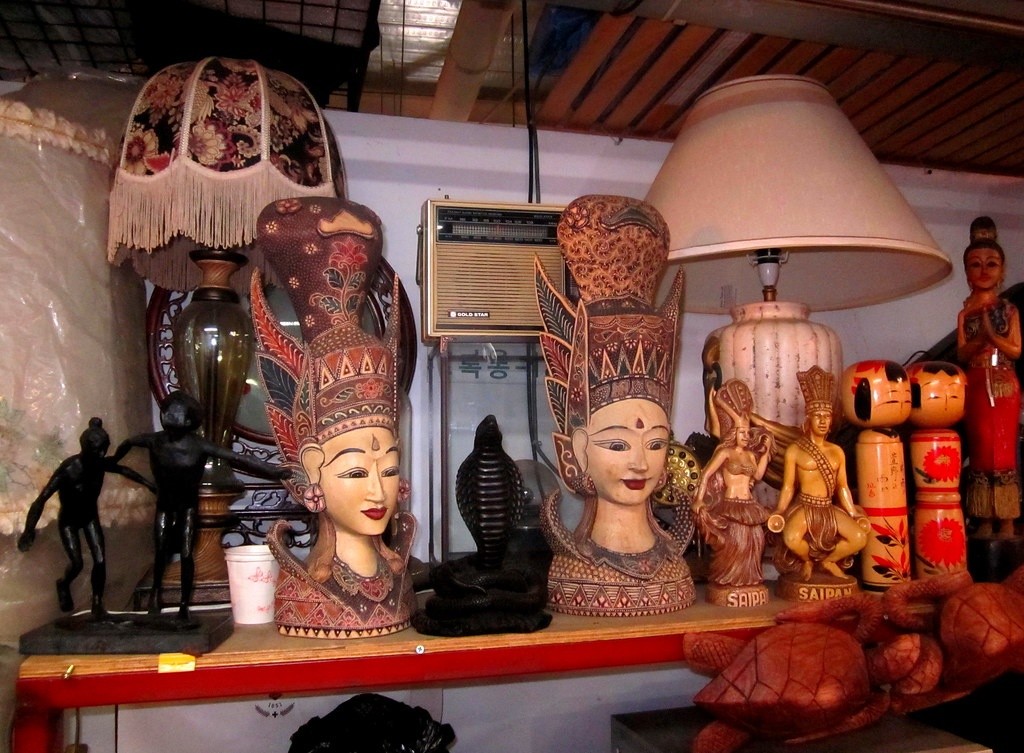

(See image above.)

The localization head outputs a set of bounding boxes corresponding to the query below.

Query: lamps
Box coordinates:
[642,75,954,583]
[107,56,346,614]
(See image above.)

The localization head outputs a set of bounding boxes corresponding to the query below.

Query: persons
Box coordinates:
[534,195,1021,617]
[248,196,419,638]
[17,391,293,629]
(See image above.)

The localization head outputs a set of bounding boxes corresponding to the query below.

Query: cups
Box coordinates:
[225,545,280,625]
